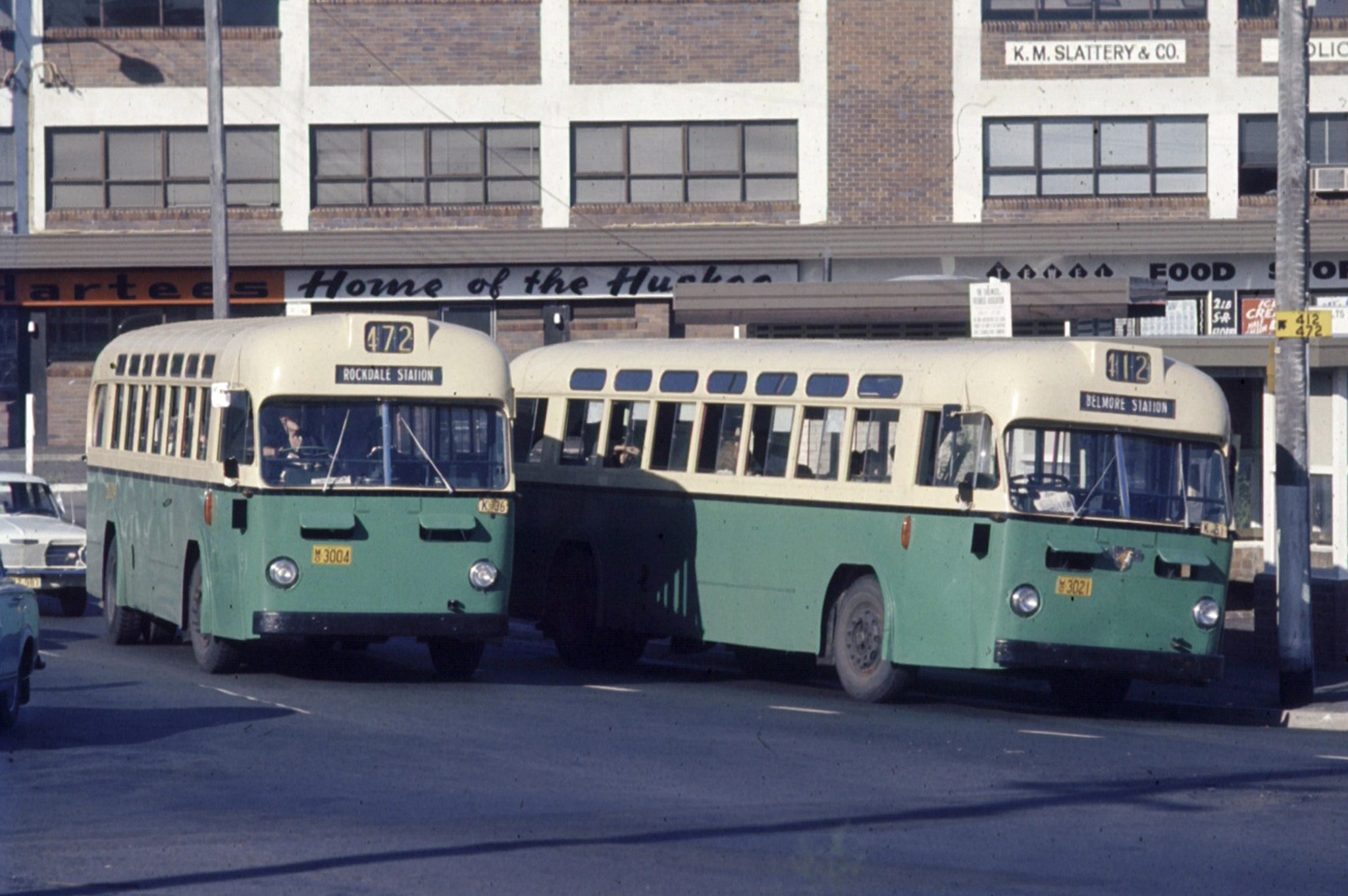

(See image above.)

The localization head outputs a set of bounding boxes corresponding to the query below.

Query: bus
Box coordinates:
[81,312,517,674]
[509,338,1237,707]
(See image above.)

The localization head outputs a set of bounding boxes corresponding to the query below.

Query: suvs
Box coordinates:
[0,470,88,615]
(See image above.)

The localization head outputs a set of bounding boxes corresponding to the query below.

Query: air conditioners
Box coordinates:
[1309,168,1348,193]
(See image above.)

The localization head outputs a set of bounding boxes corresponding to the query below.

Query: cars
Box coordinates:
[0,577,45,720]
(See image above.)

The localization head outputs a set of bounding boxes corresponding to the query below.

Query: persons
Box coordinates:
[588,424,642,466]
[718,424,763,479]
[123,390,244,469]
[250,401,340,488]
